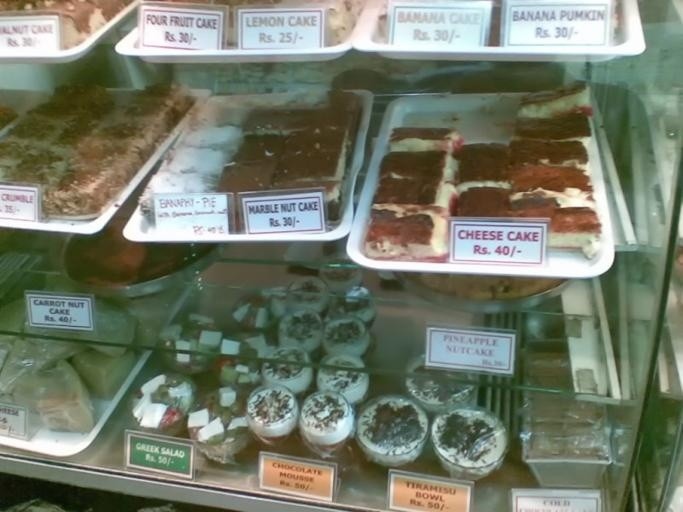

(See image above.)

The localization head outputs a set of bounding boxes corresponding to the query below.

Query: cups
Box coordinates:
[246,257,376,458]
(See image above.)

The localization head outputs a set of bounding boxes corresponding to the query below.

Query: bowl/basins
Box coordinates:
[356,392,430,468]
[430,408,510,479]
[406,355,478,413]
[129,286,286,469]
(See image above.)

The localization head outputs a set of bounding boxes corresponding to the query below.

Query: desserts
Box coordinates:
[127,258,508,490]
[365,80,601,262]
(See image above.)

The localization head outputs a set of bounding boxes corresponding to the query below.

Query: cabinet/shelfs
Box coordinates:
[0,0,683,512]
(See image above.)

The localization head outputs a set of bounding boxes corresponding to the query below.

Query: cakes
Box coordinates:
[0,0,134,50]
[0,286,179,433]
[139,88,359,232]
[1,84,192,216]
[172,0,365,46]
